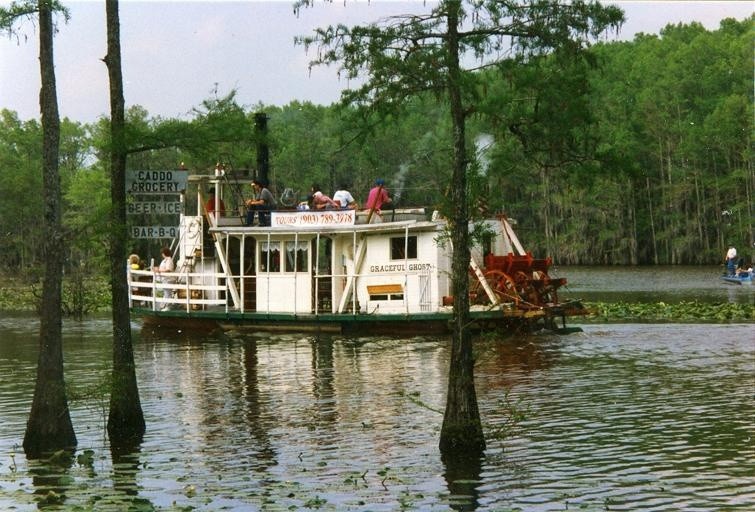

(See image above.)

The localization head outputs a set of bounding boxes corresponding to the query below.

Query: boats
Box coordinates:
[720,271,755,285]
[125,107,591,336]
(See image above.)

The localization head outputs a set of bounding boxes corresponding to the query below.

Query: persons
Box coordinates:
[153,246,176,310]
[365,177,393,210]
[132,261,152,302]
[129,254,140,291]
[206,187,225,217]
[299,184,338,211]
[735,264,753,277]
[332,183,359,210]
[725,244,737,276]
[243,180,277,226]
